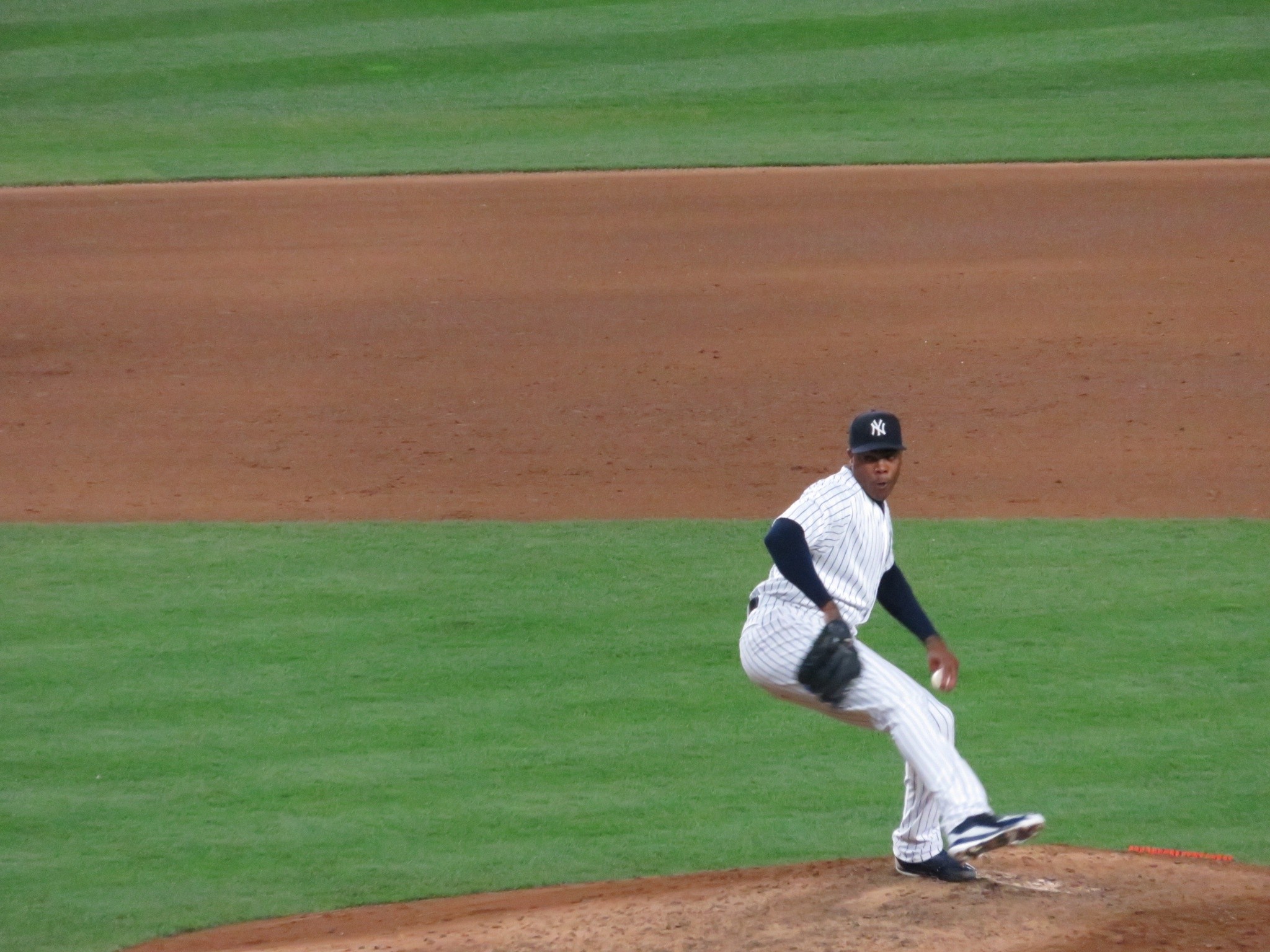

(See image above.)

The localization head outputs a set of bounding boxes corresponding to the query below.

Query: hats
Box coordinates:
[849,409,903,455]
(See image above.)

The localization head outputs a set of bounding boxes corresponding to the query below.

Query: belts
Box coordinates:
[748,597,759,614]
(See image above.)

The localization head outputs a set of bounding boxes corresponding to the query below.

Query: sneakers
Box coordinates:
[947,813,1046,863]
[895,849,977,883]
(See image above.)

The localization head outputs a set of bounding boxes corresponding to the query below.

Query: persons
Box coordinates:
[738,409,1046,884]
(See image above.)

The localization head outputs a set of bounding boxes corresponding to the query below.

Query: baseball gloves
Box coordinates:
[796,619,863,702]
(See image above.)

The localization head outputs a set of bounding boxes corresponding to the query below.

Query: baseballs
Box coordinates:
[931,668,952,690]
